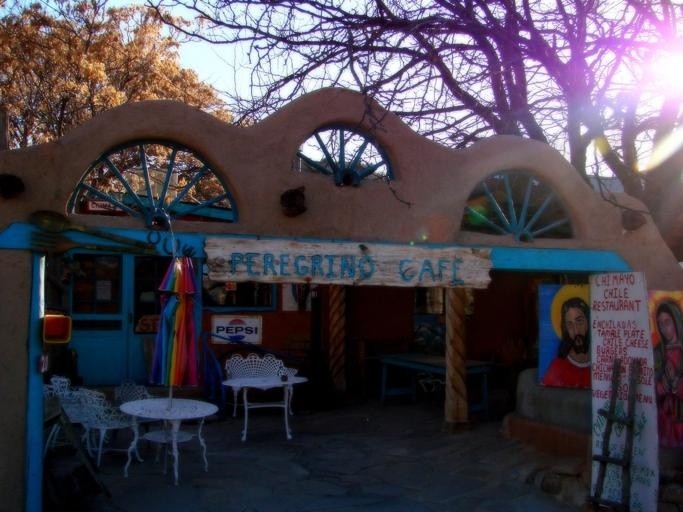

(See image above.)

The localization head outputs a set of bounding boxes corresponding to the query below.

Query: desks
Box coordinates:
[371,353,495,409]
[118,398,219,485]
[221,376,309,441]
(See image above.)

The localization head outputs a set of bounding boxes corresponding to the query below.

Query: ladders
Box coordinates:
[585,358,639,512]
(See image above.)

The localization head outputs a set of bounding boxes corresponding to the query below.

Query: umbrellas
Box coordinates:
[144,253,203,409]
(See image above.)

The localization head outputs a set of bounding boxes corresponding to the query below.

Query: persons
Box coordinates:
[655,358,683,448]
[538,295,591,388]
[653,298,682,382]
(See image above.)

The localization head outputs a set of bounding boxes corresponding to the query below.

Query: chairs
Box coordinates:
[223,352,298,418]
[44,376,156,479]
